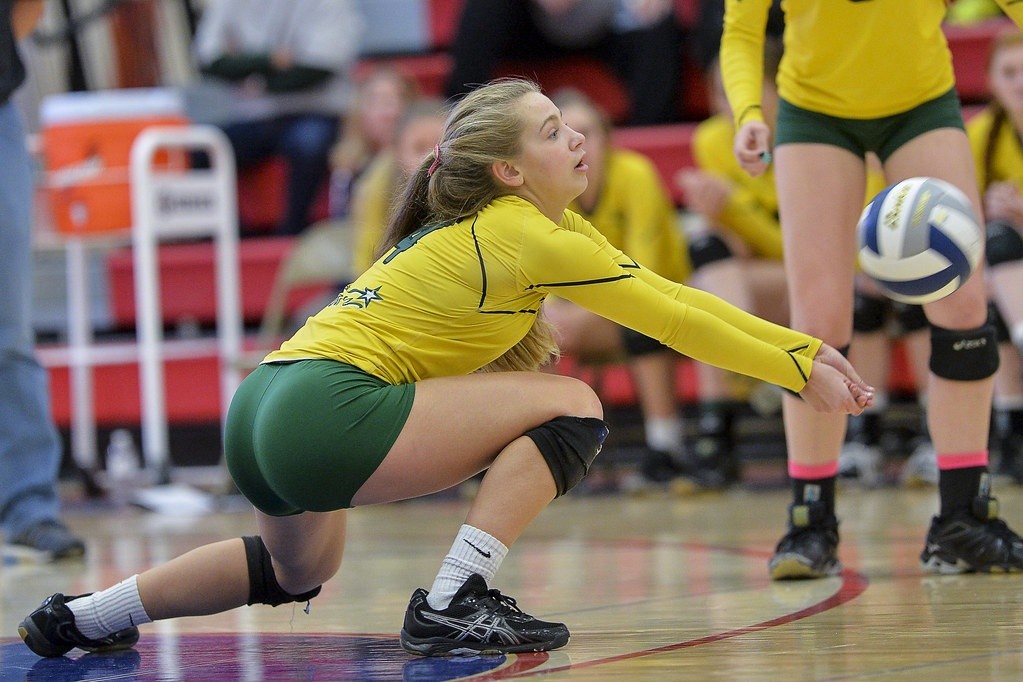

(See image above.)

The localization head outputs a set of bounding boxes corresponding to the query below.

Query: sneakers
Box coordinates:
[16,592,139,658]
[400,573,570,656]
[767,503,841,578]
[919,514,1023,575]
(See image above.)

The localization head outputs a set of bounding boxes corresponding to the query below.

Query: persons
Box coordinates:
[26,77,876,661]
[0,0,1022,569]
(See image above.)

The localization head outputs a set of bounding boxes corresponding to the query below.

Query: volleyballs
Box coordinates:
[854,177,986,307]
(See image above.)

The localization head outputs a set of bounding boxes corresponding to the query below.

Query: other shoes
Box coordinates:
[906,442,939,486]
[20,520,86,561]
[856,447,896,487]
[671,446,743,495]
[620,450,685,494]
[838,440,865,479]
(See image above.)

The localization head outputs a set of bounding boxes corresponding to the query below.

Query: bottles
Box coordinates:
[107,429,141,506]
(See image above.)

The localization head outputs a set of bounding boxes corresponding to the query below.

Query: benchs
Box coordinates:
[40,13,1023,446]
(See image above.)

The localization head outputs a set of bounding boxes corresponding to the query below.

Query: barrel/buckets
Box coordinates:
[40,86,189,240]
